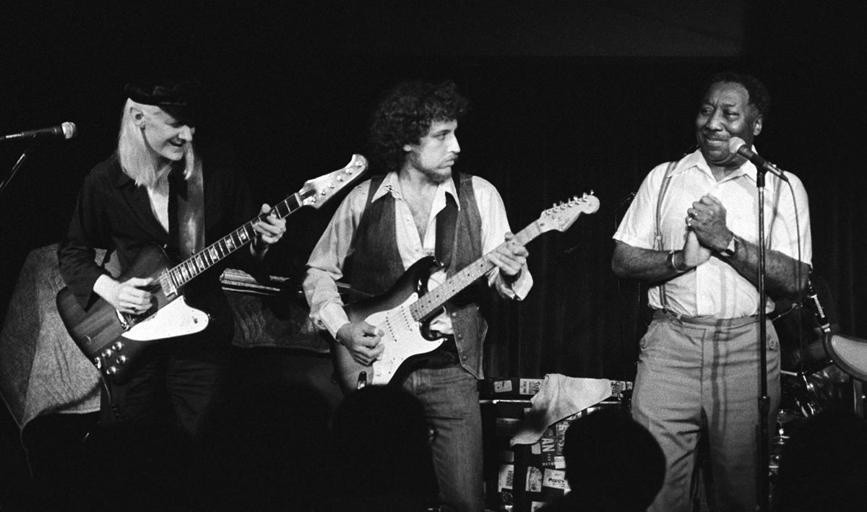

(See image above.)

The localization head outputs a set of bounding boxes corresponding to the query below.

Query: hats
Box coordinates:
[124,70,206,128]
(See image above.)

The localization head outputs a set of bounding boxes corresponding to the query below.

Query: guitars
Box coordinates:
[329,191,601,391]
[55,154,370,382]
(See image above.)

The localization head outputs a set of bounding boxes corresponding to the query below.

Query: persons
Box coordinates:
[611,73,814,512]
[58,72,286,512]
[302,84,534,512]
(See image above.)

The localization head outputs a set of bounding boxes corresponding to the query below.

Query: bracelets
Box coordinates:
[666,250,687,274]
[719,232,740,259]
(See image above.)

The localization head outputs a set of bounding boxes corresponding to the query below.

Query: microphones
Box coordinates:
[728,136,782,176]
[20,121,78,139]
